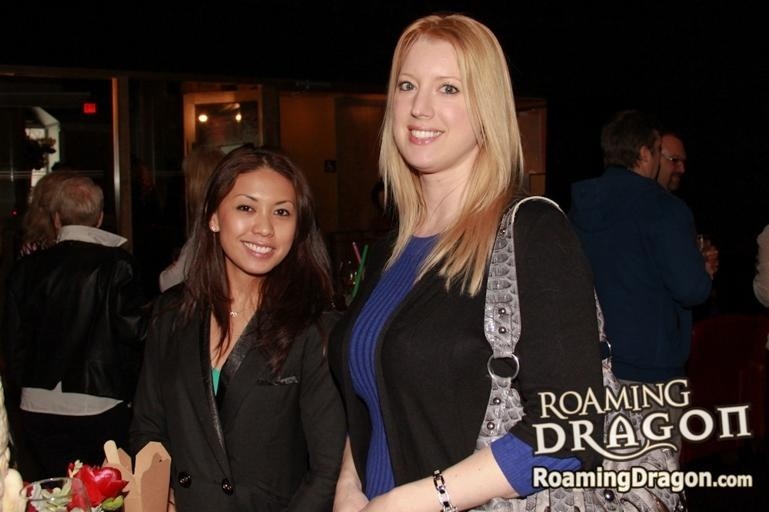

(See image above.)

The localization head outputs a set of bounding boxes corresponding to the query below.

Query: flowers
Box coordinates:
[24,460,128,512]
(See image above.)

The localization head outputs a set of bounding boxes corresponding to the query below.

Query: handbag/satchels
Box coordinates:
[467,195,690,512]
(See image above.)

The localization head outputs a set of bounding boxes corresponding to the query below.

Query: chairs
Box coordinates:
[680,314,769,471]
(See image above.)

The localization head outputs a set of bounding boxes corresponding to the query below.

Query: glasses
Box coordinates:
[661,153,688,166]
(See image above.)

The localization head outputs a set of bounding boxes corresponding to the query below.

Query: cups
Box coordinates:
[19,478,91,512]
[336,260,366,294]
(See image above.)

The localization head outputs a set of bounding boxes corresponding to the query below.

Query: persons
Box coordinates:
[326,14,604,511]
[3,100,769,511]
